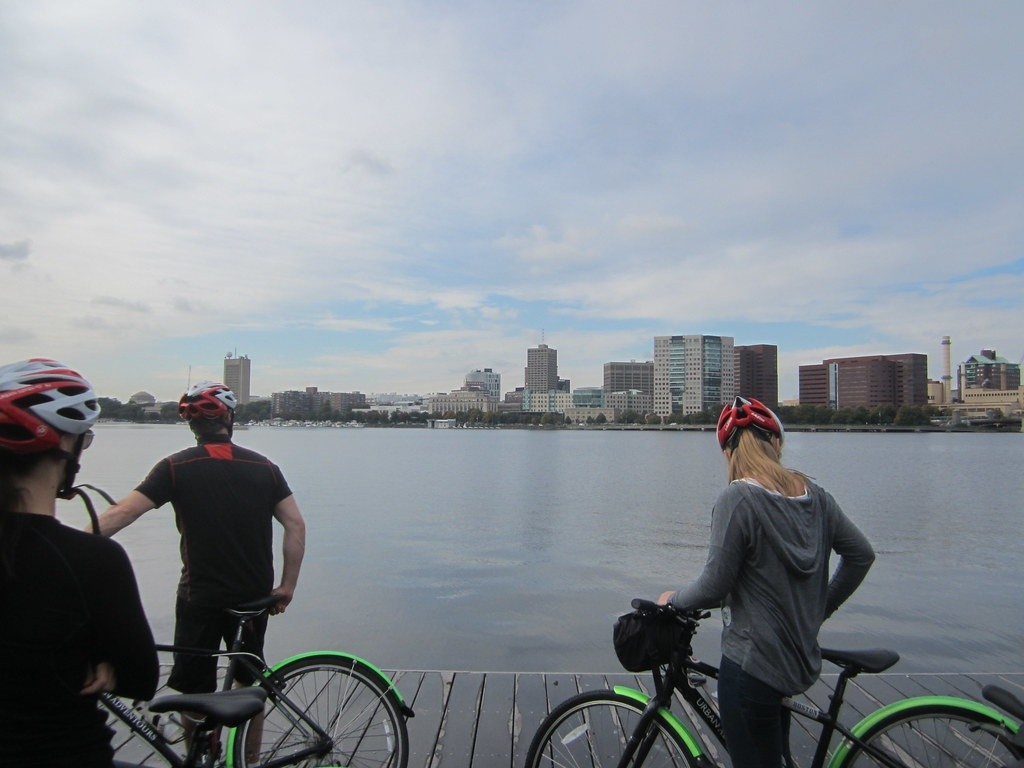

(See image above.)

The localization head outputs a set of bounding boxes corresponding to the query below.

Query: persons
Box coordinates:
[656,395,876,768]
[84,380,306,768]
[0,357,160,768]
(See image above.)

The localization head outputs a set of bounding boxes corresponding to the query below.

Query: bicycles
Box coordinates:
[524,597,1024,768]
[94,593,416,768]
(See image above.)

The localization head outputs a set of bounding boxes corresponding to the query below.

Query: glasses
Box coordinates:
[75,430,96,451]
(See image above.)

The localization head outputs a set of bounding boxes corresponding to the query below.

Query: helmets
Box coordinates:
[717,396,785,450]
[0,358,101,453]
[178,382,239,424]
